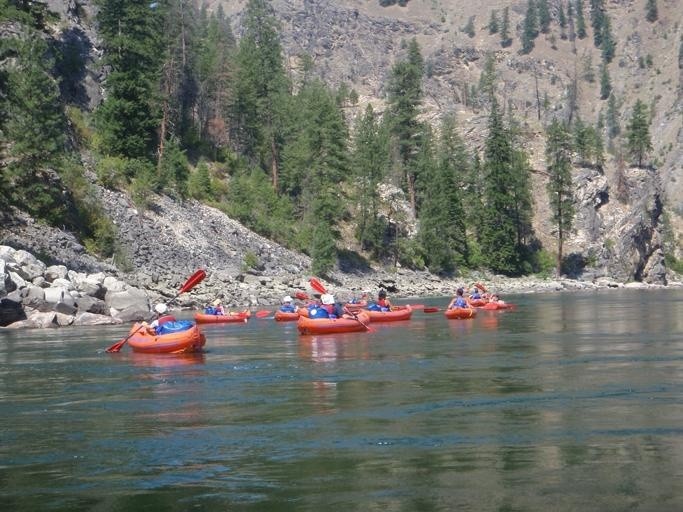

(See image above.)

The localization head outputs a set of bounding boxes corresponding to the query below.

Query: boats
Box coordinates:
[444,296,513,321]
[126,321,206,354]
[194,308,252,324]
[275,300,413,335]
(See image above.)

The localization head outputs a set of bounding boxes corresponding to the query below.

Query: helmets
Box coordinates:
[155,304,167,314]
[284,296,293,301]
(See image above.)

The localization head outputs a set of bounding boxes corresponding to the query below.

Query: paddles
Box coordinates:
[310,279,371,330]
[392,304,424,309]
[105,270,206,352]
[225,313,248,318]
[256,309,281,318]
[475,284,491,295]
[423,307,449,312]
[296,294,318,301]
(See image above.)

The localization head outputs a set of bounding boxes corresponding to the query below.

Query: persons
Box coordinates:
[214,303,224,315]
[448,287,474,309]
[488,293,499,302]
[471,288,481,298]
[280,288,392,319]
[141,303,176,333]
